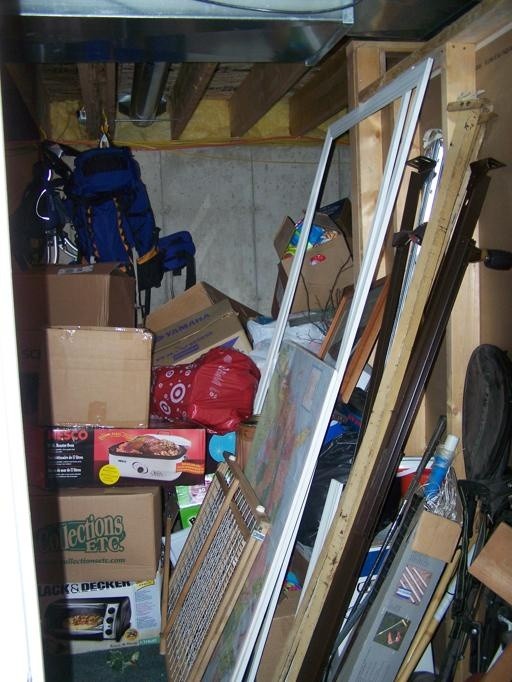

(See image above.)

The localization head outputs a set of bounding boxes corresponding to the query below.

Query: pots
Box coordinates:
[107,442,206,481]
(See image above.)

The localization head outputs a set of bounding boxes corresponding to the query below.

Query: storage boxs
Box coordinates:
[44,487,165,587]
[42,554,167,661]
[271,197,351,321]
[144,281,262,368]
[44,426,210,489]
[46,327,155,428]
[42,258,134,327]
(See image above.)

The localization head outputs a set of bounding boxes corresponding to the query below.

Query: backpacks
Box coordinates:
[42,137,164,293]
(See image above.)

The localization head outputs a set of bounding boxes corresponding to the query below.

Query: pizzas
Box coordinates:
[63,614,103,631]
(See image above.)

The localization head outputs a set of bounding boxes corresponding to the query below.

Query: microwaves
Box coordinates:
[43,596,132,642]
